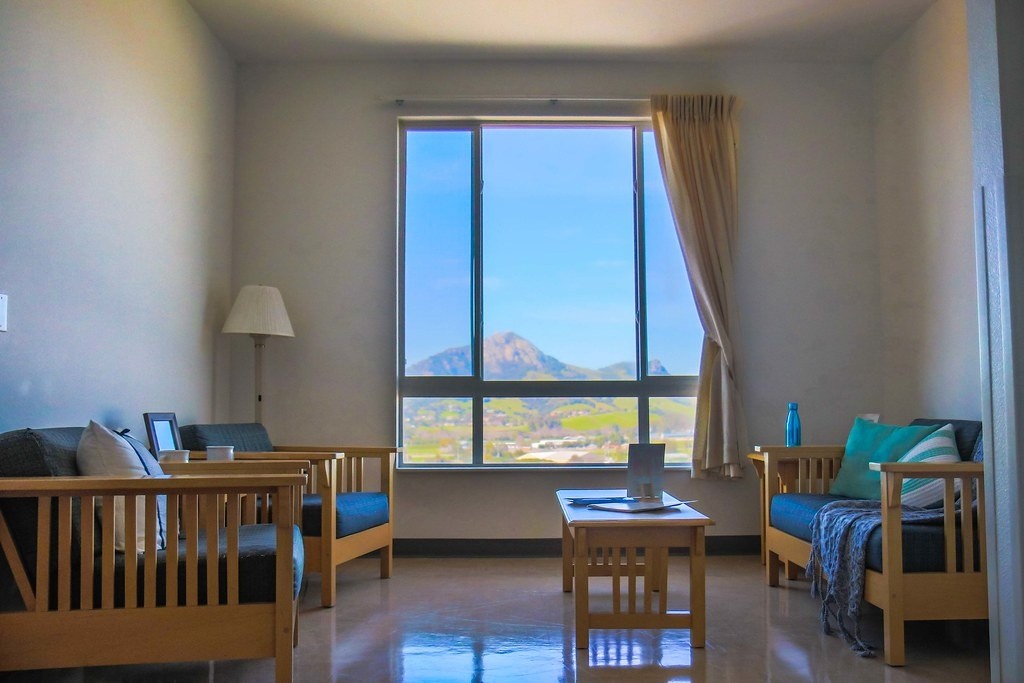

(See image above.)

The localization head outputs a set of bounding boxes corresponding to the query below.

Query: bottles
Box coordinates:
[785,403,802,447]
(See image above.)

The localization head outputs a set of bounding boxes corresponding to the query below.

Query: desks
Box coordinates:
[747,452,844,581]
[557,488,716,650]
[219,493,249,526]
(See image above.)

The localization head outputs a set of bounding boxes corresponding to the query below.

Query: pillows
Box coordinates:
[828,417,942,501]
[897,421,962,508]
[77,421,180,554]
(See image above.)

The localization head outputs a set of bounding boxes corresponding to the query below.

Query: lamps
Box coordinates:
[221,283,295,422]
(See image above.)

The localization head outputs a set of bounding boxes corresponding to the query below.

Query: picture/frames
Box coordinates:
[144,412,184,461]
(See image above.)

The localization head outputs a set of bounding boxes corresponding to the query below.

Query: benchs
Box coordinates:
[0,420,305,683]
[181,424,406,608]
[754,416,990,667]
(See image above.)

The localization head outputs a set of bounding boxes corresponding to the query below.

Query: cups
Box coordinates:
[206,446,234,460]
[158,450,190,461]
